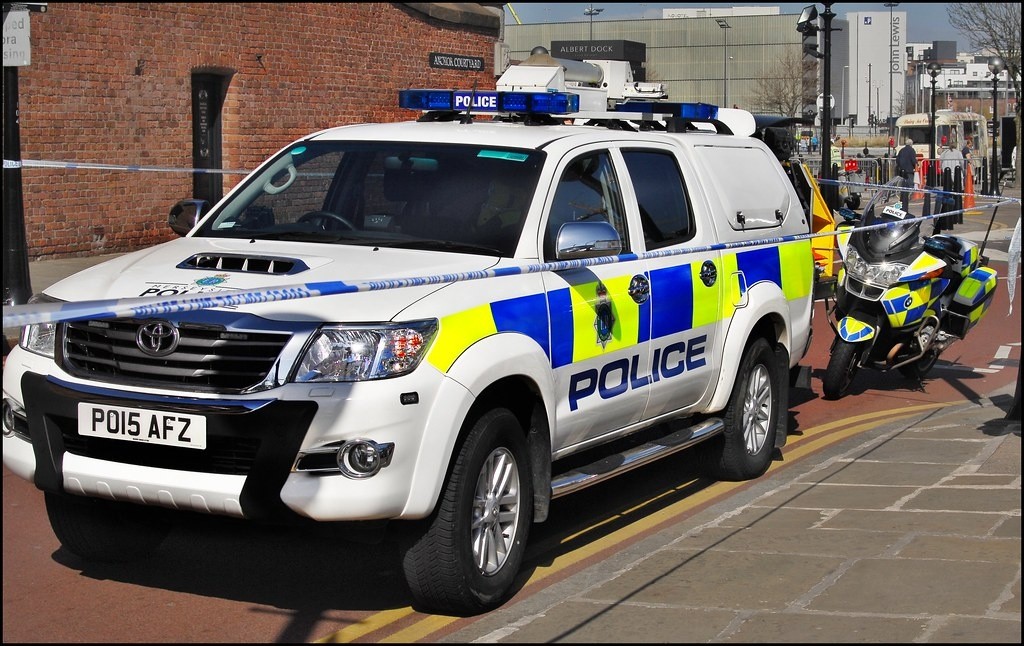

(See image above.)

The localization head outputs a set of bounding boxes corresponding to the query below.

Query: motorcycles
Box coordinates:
[821,177,997,399]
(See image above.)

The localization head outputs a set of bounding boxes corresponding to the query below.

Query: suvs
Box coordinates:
[4,61,815,615]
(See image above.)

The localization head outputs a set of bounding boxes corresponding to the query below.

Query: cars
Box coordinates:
[800,130,814,141]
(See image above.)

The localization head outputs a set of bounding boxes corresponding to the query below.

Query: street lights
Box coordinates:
[883,3,899,135]
[797,5,835,219]
[948,79,951,87]
[715,18,732,107]
[585,5,604,41]
[868,63,875,126]
[912,62,918,114]
[877,86,881,124]
[841,65,849,125]
[921,61,924,114]
[927,63,942,189]
[808,109,814,154]
[988,57,1004,196]
[729,56,733,107]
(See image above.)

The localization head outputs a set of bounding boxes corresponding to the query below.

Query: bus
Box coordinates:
[893,106,988,172]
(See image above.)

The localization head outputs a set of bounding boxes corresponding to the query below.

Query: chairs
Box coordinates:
[387,178,451,242]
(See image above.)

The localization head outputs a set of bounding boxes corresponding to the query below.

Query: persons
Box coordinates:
[801,136,818,153]
[939,143,964,192]
[896,138,916,202]
[961,139,975,194]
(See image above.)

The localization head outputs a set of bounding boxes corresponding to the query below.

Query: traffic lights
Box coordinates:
[870,114,875,128]
[851,119,854,128]
